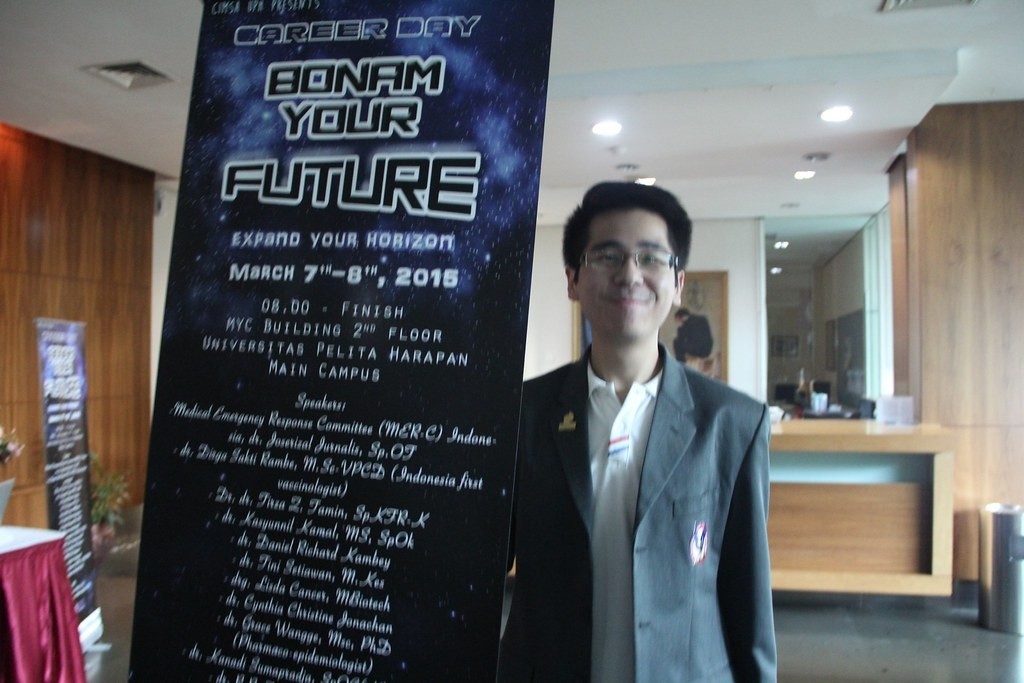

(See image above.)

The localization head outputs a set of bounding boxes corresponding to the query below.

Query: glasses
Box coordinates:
[576,245,686,272]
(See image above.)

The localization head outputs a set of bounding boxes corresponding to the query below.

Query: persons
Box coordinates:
[671,308,712,364]
[498,181,777,682]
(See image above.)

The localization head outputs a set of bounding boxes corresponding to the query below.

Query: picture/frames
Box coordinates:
[572,270,729,385]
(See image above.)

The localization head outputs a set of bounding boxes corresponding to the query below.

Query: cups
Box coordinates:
[876,396,914,427]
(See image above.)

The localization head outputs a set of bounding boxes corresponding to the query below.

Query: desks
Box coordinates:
[766,419,954,597]
[0,523,87,683]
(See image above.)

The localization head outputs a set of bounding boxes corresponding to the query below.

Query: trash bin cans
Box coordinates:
[976,502,1023,636]
